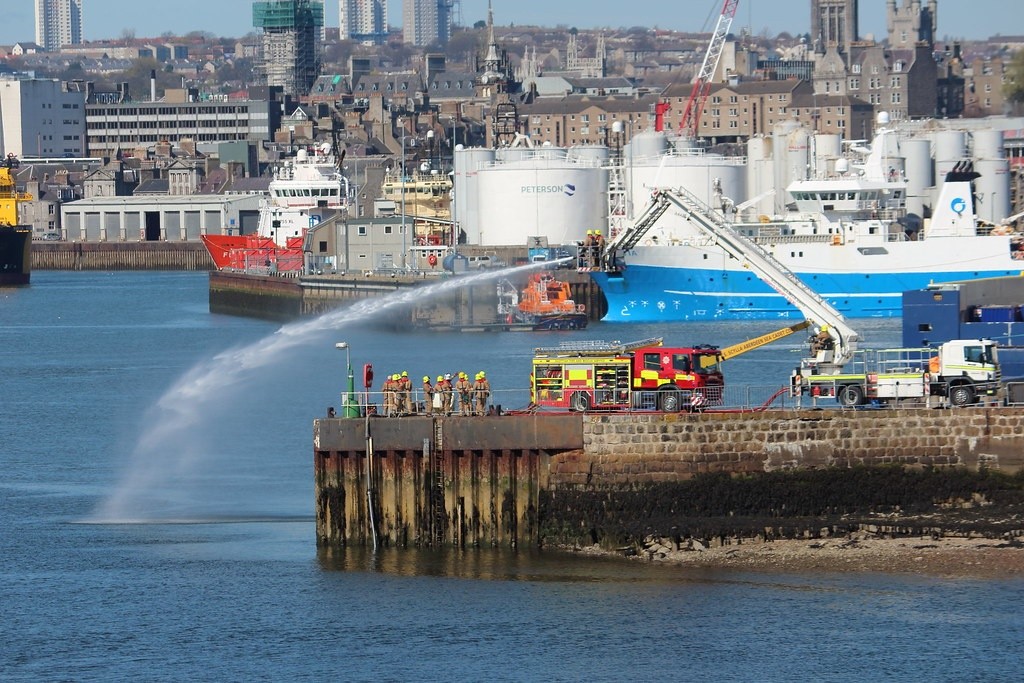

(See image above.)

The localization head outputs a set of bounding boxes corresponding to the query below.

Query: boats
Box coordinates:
[204,164,611,336]
[597,176,1024,320]
[0,147,33,290]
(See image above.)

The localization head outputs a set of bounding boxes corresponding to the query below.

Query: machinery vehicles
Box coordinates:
[577,183,1006,409]
[671,1,739,137]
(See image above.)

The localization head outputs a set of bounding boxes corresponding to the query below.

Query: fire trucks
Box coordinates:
[529,330,724,415]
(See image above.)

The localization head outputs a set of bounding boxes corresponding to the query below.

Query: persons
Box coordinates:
[810,325,831,358]
[579,229,606,268]
[382,371,490,417]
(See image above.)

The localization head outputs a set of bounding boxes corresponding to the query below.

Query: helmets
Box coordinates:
[678,356,685,360]
[397,374,401,379]
[587,230,593,235]
[821,325,828,332]
[392,374,398,380]
[422,376,429,383]
[475,374,481,380]
[437,376,444,382]
[401,370,408,377]
[480,371,485,378]
[595,230,601,235]
[458,372,464,378]
[464,374,469,380]
